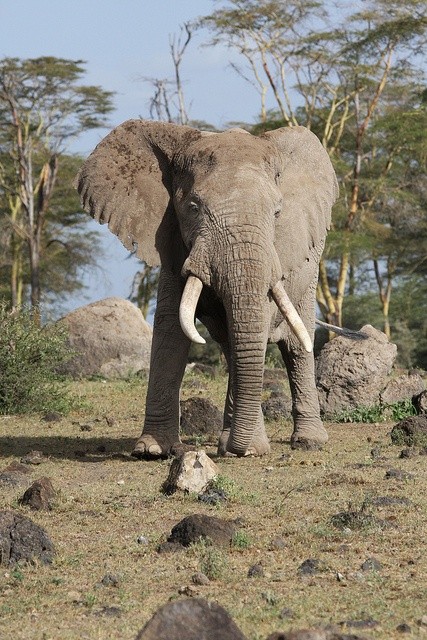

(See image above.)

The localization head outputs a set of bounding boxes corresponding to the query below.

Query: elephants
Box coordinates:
[78,119,340,457]
